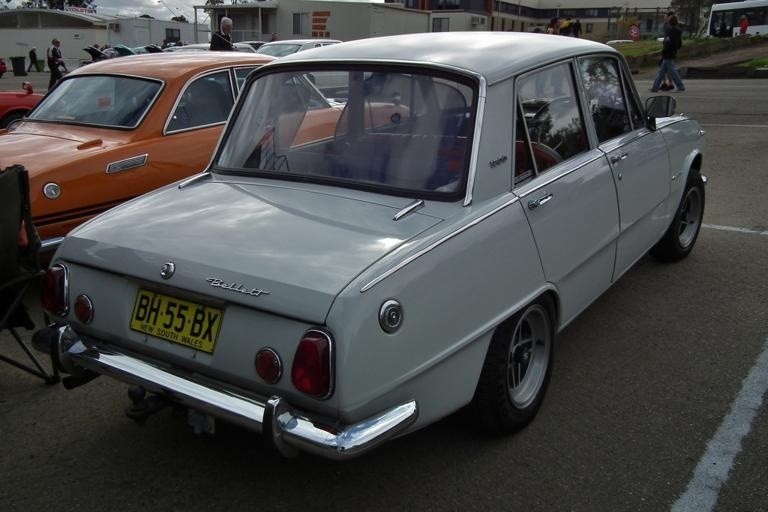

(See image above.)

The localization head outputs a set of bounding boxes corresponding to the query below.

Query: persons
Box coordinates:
[46,37,66,91]
[27,47,41,73]
[269,32,279,42]
[716,19,727,37]
[210,17,239,51]
[161,40,169,49]
[659,11,674,91]
[177,40,184,47]
[739,15,748,35]
[533,14,581,37]
[648,15,686,92]
[93,43,112,51]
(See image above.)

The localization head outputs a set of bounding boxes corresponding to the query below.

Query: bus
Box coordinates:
[706,1,768,38]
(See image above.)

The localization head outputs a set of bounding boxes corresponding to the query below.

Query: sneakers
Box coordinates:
[670,87,686,93]
[647,87,658,92]
[658,84,668,90]
[662,84,674,91]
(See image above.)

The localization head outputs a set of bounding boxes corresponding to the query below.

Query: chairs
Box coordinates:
[0,162,64,390]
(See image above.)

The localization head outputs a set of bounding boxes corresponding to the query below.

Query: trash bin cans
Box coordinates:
[34,60,44,72]
[9,56,28,76]
